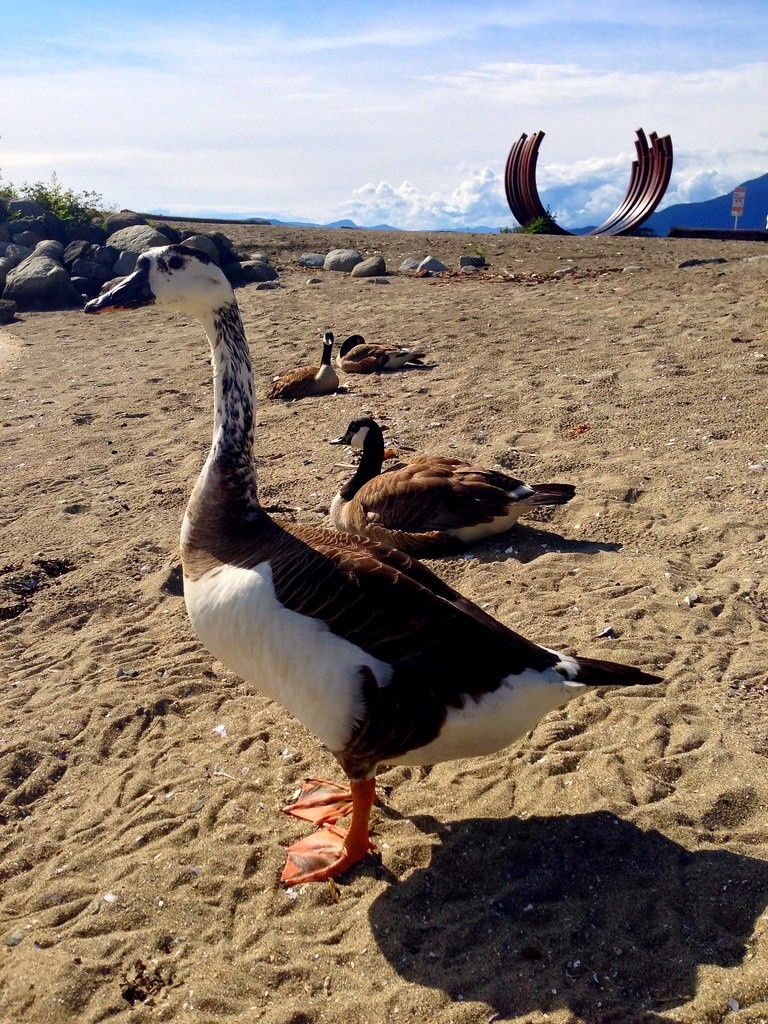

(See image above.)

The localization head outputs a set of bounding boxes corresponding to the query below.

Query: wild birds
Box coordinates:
[83,242,667,888]
